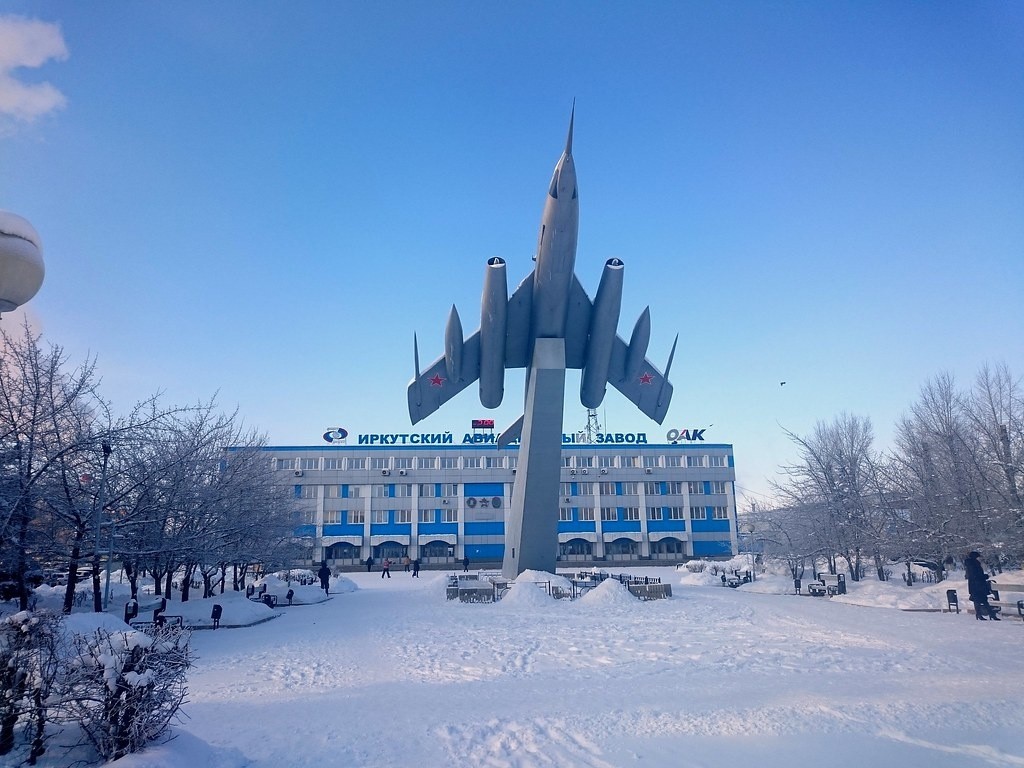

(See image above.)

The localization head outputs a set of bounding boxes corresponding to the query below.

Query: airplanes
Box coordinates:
[407,98,680,450]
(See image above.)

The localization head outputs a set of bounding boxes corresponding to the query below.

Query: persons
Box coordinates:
[965,552,1001,620]
[412,558,419,578]
[365,556,374,572]
[381,558,391,578]
[403,556,410,572]
[463,556,469,572]
[172,561,331,595]
